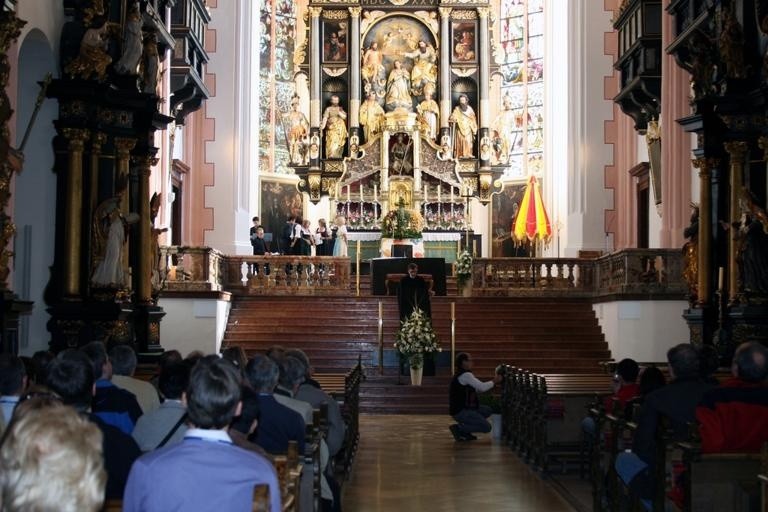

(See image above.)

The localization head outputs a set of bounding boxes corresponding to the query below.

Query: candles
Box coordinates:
[423,185,469,202]
[333,183,378,201]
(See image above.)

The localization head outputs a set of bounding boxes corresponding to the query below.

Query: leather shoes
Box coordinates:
[449,424,463,441]
[460,431,477,439]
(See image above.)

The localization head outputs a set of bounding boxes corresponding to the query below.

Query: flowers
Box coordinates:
[394,306,442,369]
[454,250,473,295]
[339,207,465,238]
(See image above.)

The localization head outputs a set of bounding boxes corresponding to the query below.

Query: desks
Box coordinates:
[385,273,435,296]
[371,257,447,296]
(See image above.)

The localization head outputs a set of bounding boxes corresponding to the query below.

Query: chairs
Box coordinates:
[391,244,413,258]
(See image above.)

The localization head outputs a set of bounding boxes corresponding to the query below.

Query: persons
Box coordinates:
[442,143,449,152]
[149,192,167,283]
[248,212,348,284]
[362,43,384,88]
[310,144,318,155]
[583,340,768,512]
[387,60,412,110]
[722,185,767,293]
[70,1,146,78]
[329,32,345,61]
[359,8,385,34]
[450,95,477,159]
[360,88,384,142]
[416,85,439,141]
[406,41,438,95]
[413,11,439,35]
[683,198,700,247]
[479,129,506,166]
[443,351,503,443]
[391,133,412,173]
[398,263,430,321]
[91,196,125,285]
[350,143,357,151]
[321,94,348,159]
[262,181,302,220]
[1,334,348,511]
[288,93,309,164]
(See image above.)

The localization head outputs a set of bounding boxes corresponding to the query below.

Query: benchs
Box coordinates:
[500,364,768,512]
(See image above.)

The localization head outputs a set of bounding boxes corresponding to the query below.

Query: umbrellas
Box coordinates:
[510,173,552,287]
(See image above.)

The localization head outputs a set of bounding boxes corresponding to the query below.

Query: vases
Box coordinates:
[409,364,423,386]
[462,278,473,297]
